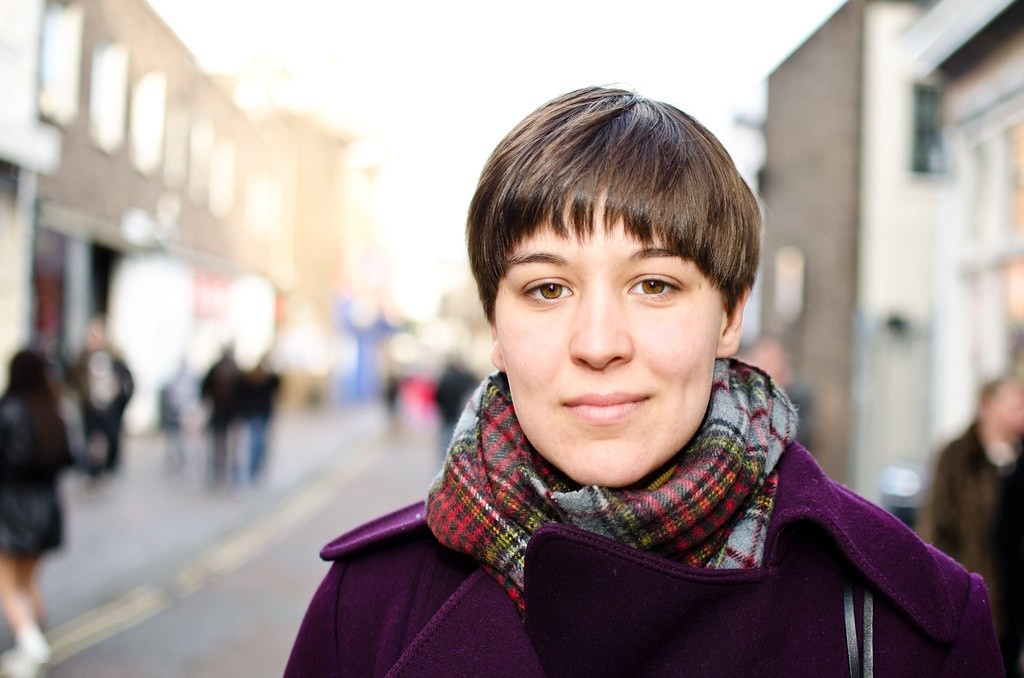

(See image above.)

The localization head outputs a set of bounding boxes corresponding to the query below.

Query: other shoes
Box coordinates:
[0,624,52,678]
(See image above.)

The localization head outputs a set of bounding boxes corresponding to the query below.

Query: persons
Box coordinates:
[0,349,73,677]
[73,316,134,486]
[927,378,1024,677]
[282,87,1007,678]
[206,338,275,491]
